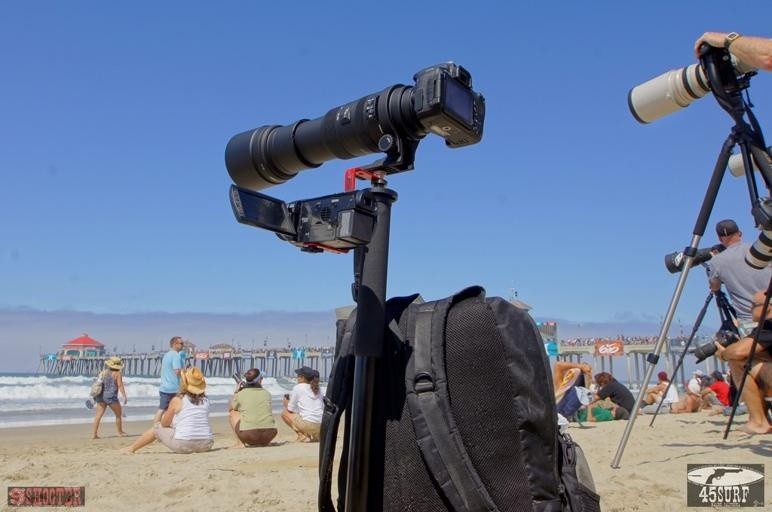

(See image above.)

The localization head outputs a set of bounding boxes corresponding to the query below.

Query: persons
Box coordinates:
[228,368,278,448]
[153,337,185,442]
[121,367,214,455]
[280,367,325,443]
[92,357,128,439]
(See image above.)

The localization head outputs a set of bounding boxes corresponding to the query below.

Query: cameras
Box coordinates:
[690,327,738,364]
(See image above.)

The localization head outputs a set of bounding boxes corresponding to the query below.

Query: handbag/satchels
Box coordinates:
[90,379,103,397]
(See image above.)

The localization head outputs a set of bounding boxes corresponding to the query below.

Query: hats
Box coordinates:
[180,367,207,396]
[658,371,667,381]
[105,356,124,370]
[710,370,723,380]
[244,368,266,384]
[295,366,314,378]
[716,219,739,237]
[692,369,703,377]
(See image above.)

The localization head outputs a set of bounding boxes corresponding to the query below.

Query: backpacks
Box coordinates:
[316,283,602,512]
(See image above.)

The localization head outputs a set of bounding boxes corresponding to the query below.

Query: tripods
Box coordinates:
[723,280,771,443]
[611,121,771,469]
[649,275,739,426]
